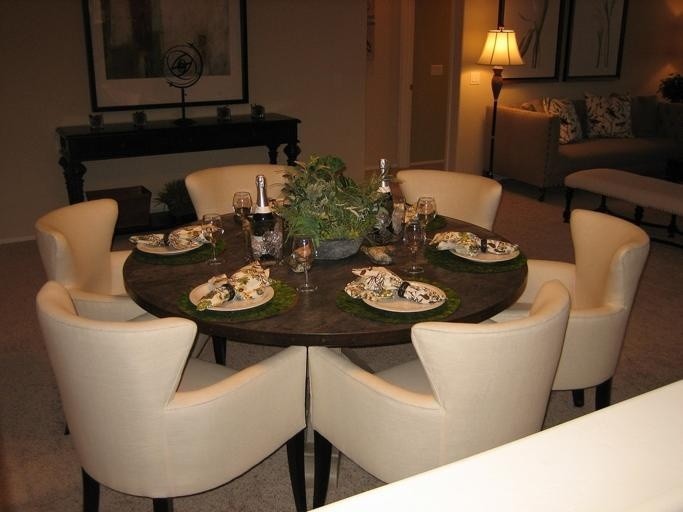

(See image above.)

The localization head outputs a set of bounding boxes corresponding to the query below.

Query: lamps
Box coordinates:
[477,25,526,184]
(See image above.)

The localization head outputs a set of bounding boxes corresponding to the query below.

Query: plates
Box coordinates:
[135,238,204,256]
[361,280,447,313]
[189,282,275,311]
[450,238,521,265]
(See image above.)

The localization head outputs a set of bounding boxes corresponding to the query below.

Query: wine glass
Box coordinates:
[403,220,426,273]
[200,191,319,294]
[416,194,438,244]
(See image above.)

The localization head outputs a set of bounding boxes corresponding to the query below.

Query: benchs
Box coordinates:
[562,167,683,248]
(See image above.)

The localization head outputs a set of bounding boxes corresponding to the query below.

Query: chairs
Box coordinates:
[308,278,571,509]
[33,197,216,434]
[184,164,303,222]
[34,277,307,512]
[395,168,503,233]
[475,206,650,409]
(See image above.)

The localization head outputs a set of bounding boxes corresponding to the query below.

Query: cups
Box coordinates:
[250,103,266,119]
[217,105,232,120]
[87,114,105,129]
[133,111,148,127]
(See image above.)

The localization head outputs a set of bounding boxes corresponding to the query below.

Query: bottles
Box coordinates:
[372,158,396,245]
[249,175,279,265]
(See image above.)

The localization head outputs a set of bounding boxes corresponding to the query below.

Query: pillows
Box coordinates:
[538,95,583,146]
[581,88,635,139]
[520,100,539,112]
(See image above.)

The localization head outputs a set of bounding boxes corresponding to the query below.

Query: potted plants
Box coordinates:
[152,178,195,217]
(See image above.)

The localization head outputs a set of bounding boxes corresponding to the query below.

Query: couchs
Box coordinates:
[484,98,683,202]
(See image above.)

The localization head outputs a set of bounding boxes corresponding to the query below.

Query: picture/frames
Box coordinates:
[565,1,629,82]
[82,0,249,113]
[495,0,563,83]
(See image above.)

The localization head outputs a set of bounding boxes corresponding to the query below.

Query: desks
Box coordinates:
[56,112,302,237]
